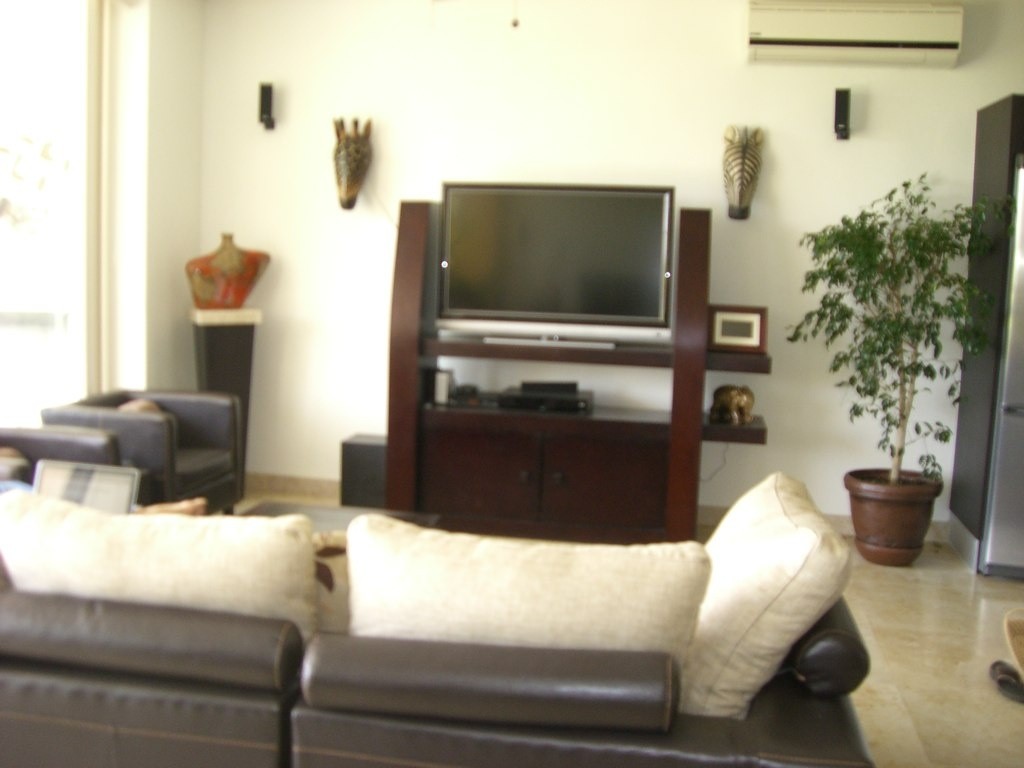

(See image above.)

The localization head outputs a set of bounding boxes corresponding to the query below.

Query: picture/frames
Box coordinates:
[709,304,768,352]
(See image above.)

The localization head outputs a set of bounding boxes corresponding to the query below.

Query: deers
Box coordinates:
[332,118,372,209]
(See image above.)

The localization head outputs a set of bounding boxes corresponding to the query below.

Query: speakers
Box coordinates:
[835,89,850,139]
[259,83,274,129]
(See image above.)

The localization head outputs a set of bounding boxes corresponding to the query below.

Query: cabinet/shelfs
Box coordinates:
[419,400,670,536]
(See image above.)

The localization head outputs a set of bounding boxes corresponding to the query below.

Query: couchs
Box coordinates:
[0,561,882,768]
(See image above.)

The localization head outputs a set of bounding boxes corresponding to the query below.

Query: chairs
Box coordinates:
[0,389,245,515]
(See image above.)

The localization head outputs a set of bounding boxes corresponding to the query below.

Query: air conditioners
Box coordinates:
[732,0,965,70]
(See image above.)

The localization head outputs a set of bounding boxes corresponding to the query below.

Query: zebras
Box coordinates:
[722,124,764,219]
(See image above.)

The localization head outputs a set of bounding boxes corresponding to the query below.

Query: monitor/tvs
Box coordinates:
[436,182,676,350]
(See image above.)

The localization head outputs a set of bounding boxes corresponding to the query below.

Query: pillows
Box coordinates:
[313,529,348,634]
[344,513,711,680]
[678,471,856,722]
[0,483,317,635]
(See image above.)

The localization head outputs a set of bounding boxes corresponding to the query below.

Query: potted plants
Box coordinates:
[785,170,1018,570]
